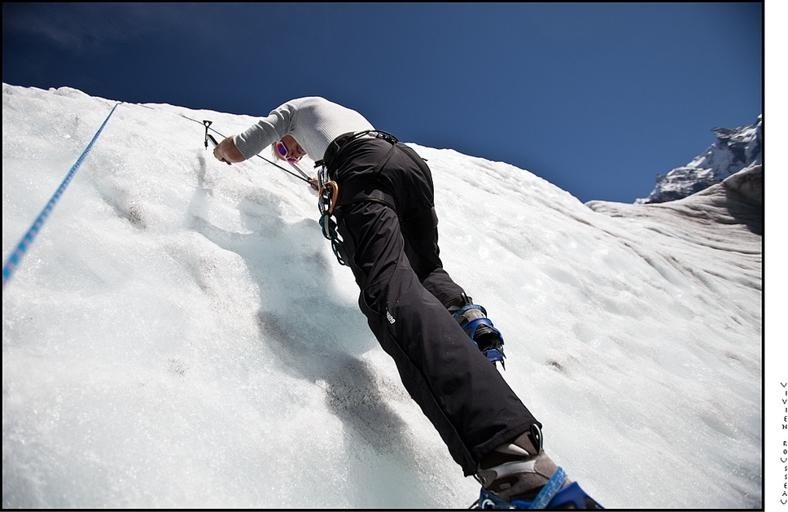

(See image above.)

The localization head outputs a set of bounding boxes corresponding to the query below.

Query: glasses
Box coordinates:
[275,142,303,165]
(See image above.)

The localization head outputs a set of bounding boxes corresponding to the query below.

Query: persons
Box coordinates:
[213,97,603,511]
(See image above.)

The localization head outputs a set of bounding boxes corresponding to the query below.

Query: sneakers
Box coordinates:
[455,304,502,350]
[477,424,600,512]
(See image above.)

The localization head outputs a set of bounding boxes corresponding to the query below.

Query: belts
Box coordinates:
[325,133,377,167]
